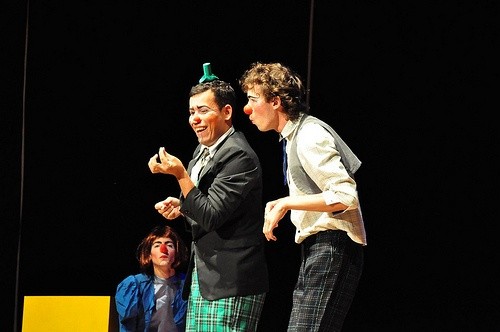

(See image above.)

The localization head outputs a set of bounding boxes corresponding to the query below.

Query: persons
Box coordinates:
[115,226,190,332]
[239,62,367,331]
[148,63,273,332]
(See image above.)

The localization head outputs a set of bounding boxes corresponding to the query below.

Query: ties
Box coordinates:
[281,135,288,186]
